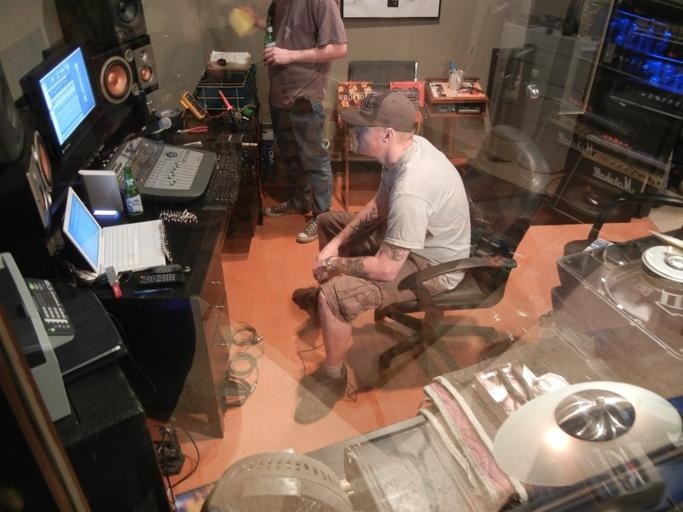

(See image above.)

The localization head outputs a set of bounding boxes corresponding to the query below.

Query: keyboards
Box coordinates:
[204,155,240,202]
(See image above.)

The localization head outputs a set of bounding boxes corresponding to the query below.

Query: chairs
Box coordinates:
[343,60,419,212]
[374,125,552,370]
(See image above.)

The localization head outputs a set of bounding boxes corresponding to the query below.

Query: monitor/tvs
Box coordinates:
[19,45,95,162]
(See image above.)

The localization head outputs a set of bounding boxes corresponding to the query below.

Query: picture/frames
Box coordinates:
[341,0,441,19]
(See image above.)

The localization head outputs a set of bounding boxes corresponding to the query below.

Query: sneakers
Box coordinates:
[292,286,320,310]
[263,200,310,217]
[297,216,318,243]
[293,363,347,424]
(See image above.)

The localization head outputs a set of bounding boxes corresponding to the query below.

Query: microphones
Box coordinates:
[134,116,172,137]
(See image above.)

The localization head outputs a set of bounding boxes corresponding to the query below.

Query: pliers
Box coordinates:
[177,126,209,133]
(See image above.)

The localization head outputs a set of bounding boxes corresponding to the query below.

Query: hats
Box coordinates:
[340,88,416,132]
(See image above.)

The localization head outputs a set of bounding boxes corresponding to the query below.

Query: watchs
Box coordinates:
[321,255,336,273]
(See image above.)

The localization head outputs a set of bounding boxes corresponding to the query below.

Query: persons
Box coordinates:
[235,0,348,244]
[289,90,473,425]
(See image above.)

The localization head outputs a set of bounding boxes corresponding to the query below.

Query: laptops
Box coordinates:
[63,186,169,271]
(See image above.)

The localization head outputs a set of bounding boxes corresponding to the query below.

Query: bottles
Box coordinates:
[122,166,145,221]
[264,16,277,62]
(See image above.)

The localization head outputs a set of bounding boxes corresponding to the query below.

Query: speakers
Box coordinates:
[0,61,25,161]
[55,0,146,56]
[0,127,54,245]
[555,149,665,227]
[42,35,159,110]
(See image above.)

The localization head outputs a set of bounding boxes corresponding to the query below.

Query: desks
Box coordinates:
[424,77,489,167]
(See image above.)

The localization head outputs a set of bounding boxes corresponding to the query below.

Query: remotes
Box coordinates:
[139,274,175,284]
[149,266,180,275]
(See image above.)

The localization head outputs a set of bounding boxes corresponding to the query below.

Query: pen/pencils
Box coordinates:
[134,288,172,294]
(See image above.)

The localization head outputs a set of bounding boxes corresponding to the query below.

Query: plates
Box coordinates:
[642,244,683,284]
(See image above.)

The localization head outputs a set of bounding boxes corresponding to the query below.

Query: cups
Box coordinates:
[448,70,465,89]
